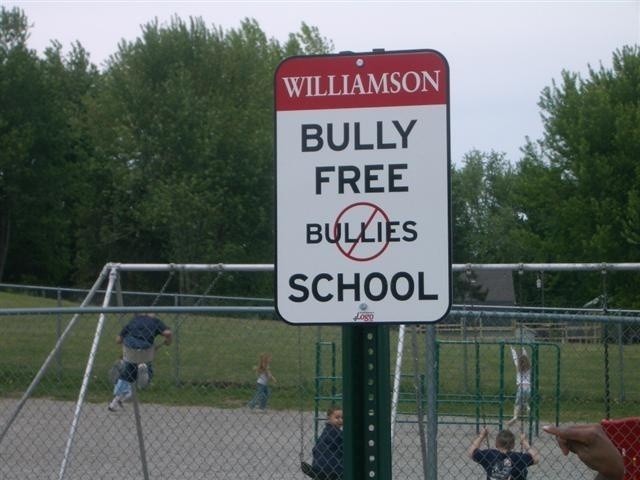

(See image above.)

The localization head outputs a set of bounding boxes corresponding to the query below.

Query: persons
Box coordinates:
[245,354,277,413]
[506,345,532,425]
[310,406,343,480]
[541,423,625,480]
[107,313,173,411]
[466,426,539,480]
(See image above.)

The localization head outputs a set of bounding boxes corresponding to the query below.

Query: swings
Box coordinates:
[297,324,346,480]
[471,317,527,480]
[121,269,225,364]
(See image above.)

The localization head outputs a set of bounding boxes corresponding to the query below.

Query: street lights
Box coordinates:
[453,271,488,393]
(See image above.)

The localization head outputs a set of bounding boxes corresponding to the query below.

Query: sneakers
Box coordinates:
[106,400,125,413]
[136,363,150,393]
[246,404,268,413]
[108,359,125,385]
[507,417,517,427]
